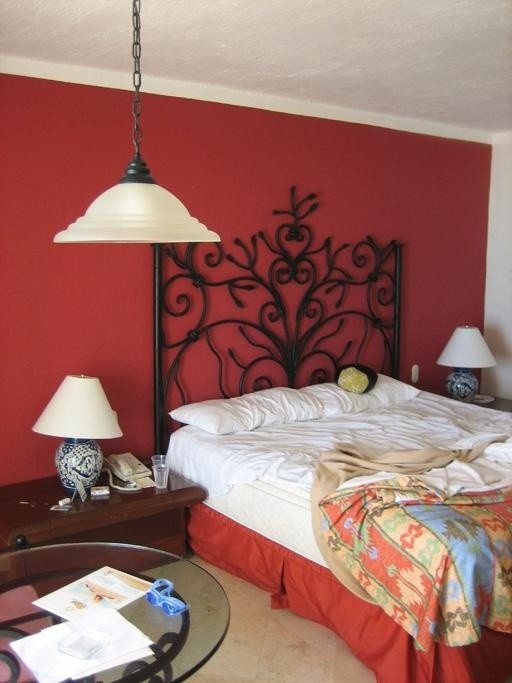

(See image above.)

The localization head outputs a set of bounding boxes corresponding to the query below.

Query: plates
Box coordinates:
[473,394,495,404]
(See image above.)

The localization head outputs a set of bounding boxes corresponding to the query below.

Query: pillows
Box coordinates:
[166,368,422,436]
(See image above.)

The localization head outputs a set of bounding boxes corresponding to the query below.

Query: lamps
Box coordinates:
[27,371,122,493]
[438,327,495,401]
[49,0,224,242]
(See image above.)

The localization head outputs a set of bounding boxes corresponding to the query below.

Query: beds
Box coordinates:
[168,386,512,681]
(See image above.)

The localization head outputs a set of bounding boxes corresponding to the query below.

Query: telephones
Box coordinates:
[103,451,153,482]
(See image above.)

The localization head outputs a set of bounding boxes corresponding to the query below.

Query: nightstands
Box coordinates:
[0,456,204,591]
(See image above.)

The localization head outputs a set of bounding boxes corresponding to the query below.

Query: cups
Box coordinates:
[150,453,169,489]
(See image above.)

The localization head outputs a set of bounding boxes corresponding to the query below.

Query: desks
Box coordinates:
[1,541,230,682]
